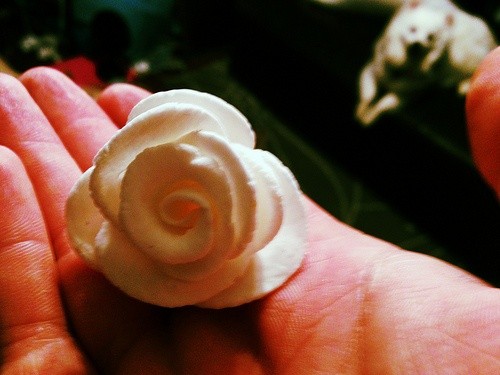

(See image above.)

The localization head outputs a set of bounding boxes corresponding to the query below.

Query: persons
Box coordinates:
[1,67,500,374]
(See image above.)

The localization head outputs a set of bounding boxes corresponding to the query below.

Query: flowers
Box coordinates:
[62,86,314,314]
